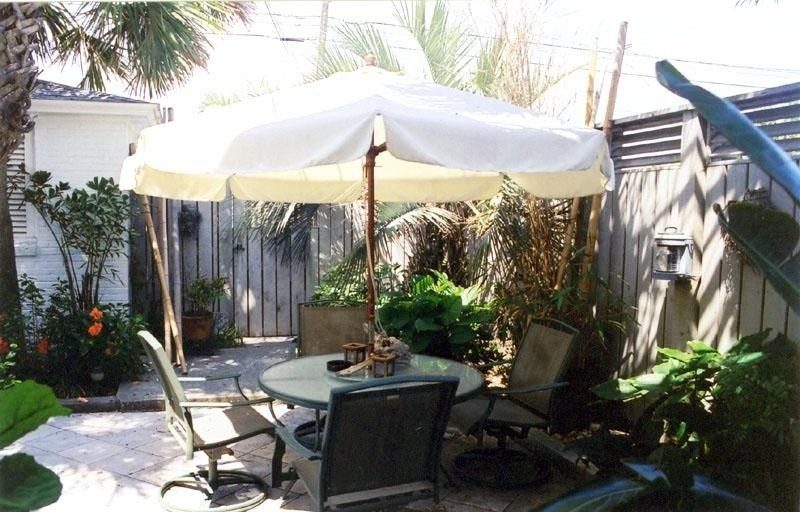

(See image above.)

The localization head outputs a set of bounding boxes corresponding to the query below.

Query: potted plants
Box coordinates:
[181,272,235,344]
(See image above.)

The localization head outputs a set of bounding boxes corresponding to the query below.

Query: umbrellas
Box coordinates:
[117,49,618,356]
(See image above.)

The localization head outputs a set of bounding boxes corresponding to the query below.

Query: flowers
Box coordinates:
[1,304,123,380]
